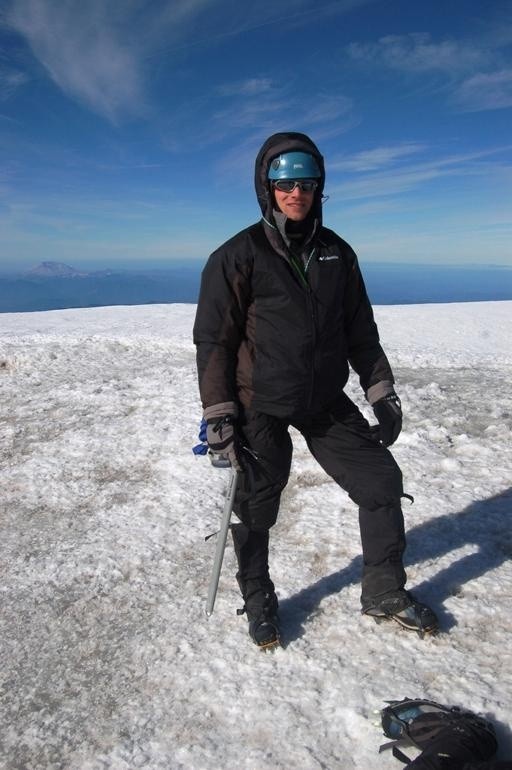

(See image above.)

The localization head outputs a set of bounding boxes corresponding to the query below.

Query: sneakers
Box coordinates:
[236,568,282,650]
[379,696,498,762]
[359,586,440,635]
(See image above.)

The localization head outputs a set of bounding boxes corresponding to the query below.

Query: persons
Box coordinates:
[192,130,439,650]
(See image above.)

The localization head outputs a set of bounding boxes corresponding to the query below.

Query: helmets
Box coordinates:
[265,149,322,181]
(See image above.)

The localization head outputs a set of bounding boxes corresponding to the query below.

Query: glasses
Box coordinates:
[270,179,319,193]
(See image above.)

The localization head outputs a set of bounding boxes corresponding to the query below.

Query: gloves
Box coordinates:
[202,401,245,474]
[365,378,404,448]
